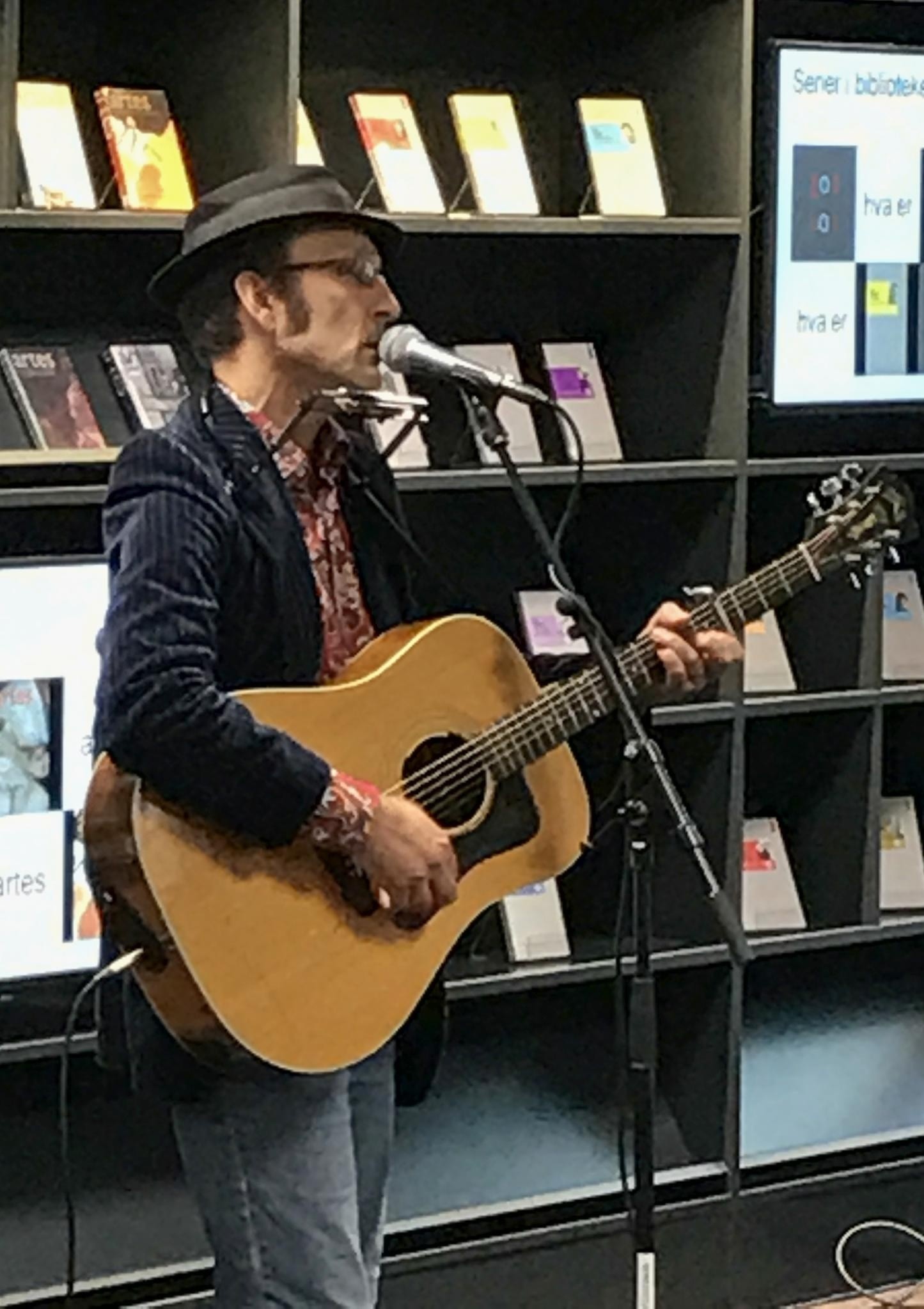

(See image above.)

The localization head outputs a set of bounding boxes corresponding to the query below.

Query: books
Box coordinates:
[516,590,589,654]
[294,99,323,164]
[338,362,429,469]
[349,95,446,214]
[881,570,924,680]
[879,798,924,908]
[100,344,191,429]
[499,878,570,963]
[453,344,542,463]
[95,88,194,210]
[16,82,95,210]
[538,342,623,461]
[0,347,105,449]
[744,608,797,692]
[448,94,539,214]
[577,99,666,216]
[739,818,805,930]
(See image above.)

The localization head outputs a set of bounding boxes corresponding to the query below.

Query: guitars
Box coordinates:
[85,463,921,1083]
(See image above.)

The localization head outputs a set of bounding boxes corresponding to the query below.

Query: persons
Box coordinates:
[0,681,51,812]
[97,163,747,1309]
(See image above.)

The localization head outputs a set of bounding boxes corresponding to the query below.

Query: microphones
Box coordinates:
[378,323,552,406]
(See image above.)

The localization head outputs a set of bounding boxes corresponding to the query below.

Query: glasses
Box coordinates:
[275,251,380,289]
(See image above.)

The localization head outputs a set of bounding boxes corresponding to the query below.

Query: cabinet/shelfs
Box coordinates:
[0,0,924,1309]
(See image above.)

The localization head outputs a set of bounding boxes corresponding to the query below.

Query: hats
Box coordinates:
[143,163,410,310]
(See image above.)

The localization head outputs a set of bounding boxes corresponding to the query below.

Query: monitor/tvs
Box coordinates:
[758,36,923,415]
